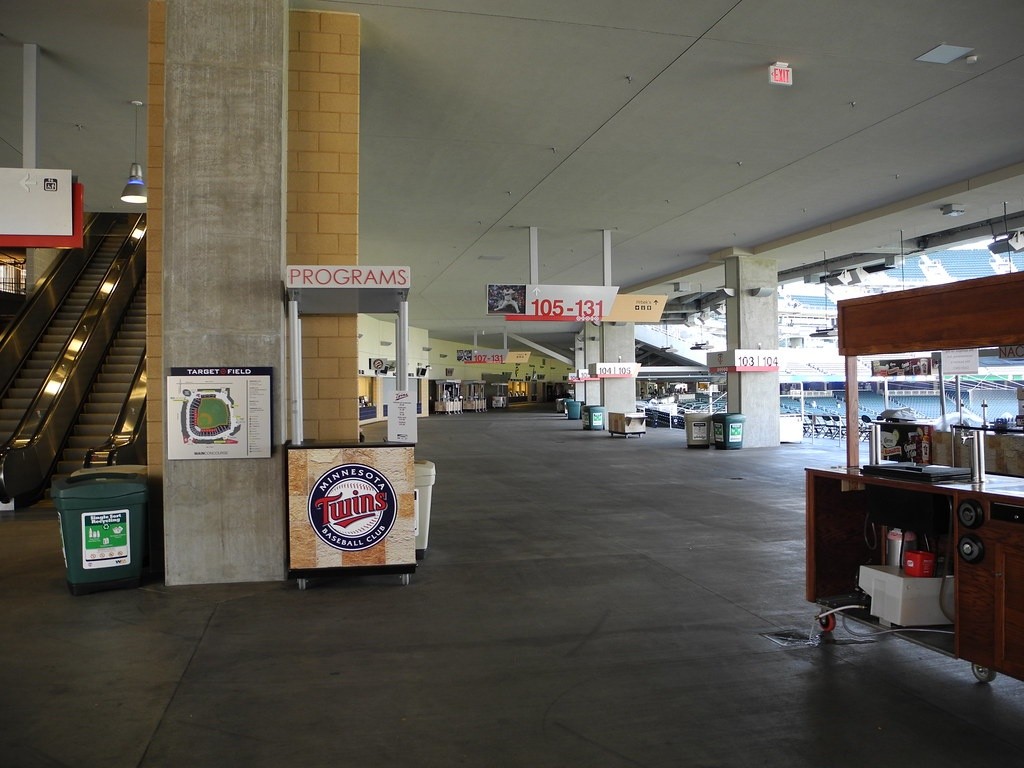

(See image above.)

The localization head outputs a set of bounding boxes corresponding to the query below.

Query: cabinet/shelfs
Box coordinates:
[804,462,1024,685]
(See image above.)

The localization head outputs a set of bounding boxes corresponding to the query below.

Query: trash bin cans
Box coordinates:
[414,459,437,560]
[580,404,605,431]
[51,463,149,595]
[711,413,745,450]
[565,400,582,420]
[683,412,711,448]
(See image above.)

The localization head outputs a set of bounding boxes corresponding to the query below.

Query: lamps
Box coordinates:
[989,227,1024,254]
[824,267,870,286]
[120,100,148,203]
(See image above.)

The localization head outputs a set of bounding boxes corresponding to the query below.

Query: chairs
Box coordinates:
[859,415,883,442]
[806,414,847,438]
